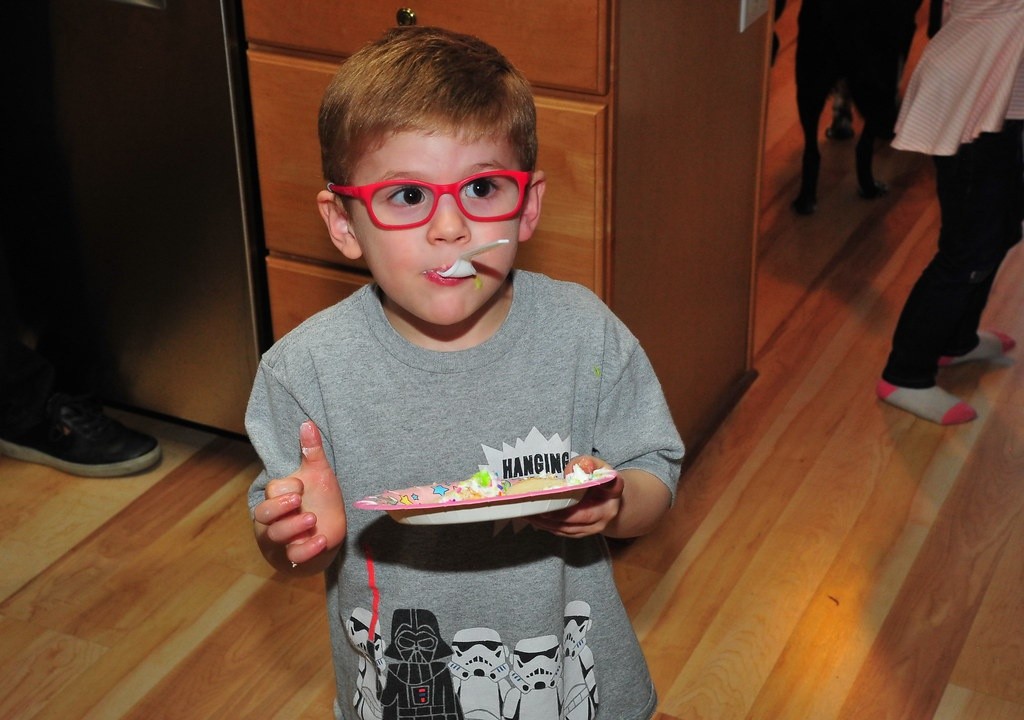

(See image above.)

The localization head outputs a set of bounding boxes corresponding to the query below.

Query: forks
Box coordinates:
[434,238,510,278]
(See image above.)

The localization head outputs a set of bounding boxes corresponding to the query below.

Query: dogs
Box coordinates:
[788,0,924,217]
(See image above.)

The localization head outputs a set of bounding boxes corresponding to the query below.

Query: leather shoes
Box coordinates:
[1,393,163,480]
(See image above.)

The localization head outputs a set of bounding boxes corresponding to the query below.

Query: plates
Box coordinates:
[354,463,620,528]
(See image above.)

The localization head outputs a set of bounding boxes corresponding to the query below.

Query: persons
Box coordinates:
[243,19,685,720]
[0,0,166,476]
[866,0,1023,430]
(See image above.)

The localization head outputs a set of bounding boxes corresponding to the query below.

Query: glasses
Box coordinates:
[327,169,536,230]
